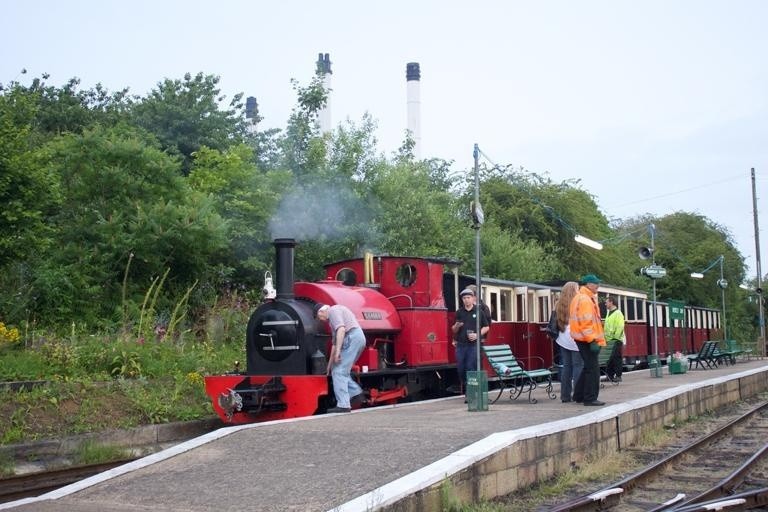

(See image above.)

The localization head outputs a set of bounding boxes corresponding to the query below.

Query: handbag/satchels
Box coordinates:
[548,311,559,340]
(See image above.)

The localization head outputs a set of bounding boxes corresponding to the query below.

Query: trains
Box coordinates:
[204,238,721,425]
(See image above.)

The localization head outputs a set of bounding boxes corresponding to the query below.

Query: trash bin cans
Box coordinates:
[648,355,663,378]
[466,371,489,410]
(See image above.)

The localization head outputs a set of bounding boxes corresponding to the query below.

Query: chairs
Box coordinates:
[687,340,745,371]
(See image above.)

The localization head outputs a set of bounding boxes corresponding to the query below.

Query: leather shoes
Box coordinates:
[327,393,367,412]
[576,399,605,407]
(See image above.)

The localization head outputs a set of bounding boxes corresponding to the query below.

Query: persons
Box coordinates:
[600,296,625,382]
[451,289,490,404]
[312,303,367,414]
[452,284,492,369]
[554,281,584,403]
[569,274,607,406]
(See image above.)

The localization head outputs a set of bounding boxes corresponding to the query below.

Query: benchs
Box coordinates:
[483,344,558,404]
[599,340,618,390]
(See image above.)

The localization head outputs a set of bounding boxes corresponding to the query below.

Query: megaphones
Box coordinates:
[755,287,763,295]
[638,246,654,261]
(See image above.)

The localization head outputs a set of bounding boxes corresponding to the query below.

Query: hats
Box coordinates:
[582,274,602,285]
[460,285,476,296]
[313,303,323,319]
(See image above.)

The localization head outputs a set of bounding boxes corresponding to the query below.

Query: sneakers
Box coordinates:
[561,398,572,402]
[600,376,622,382]
